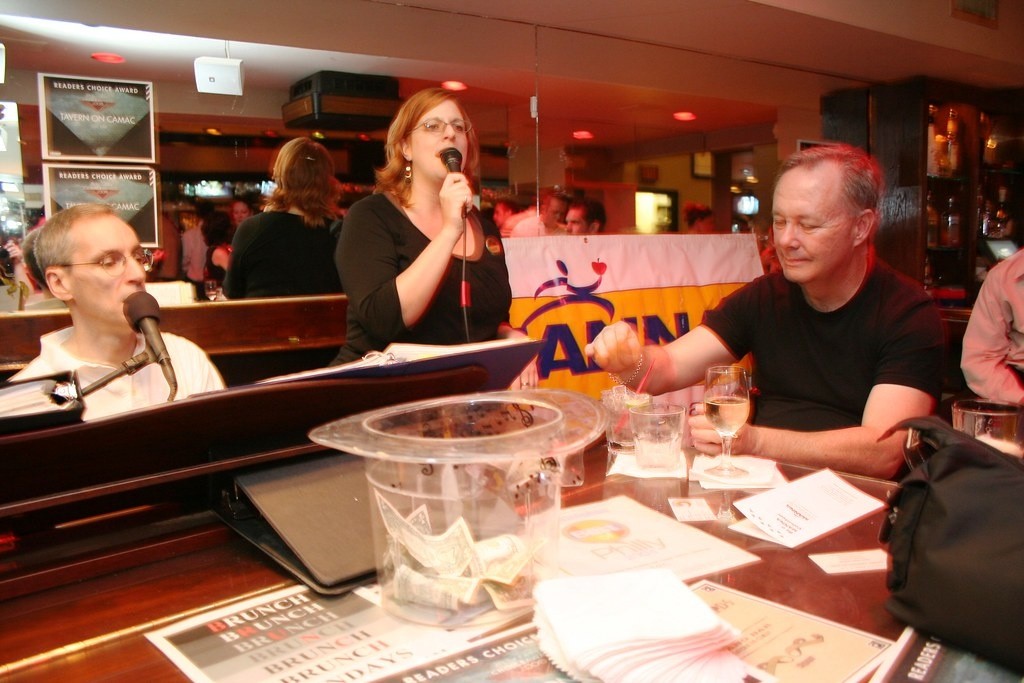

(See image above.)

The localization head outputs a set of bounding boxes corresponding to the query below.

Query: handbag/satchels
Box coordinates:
[876,416,1024,674]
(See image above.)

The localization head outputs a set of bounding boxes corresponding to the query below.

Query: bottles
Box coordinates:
[923,105,1013,296]
[0,245,15,278]
[214,288,226,301]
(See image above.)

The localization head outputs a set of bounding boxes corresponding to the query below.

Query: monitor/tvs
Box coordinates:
[978,237,1019,267]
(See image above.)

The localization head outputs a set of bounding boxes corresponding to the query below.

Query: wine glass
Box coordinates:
[702,365,752,479]
[204,281,217,301]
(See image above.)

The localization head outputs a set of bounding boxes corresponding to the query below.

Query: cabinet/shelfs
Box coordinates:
[867,75,1024,308]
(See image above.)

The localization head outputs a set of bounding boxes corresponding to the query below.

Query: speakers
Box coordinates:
[194,56,245,96]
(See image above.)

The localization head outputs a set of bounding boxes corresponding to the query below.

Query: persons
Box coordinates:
[329,88,527,372]
[960,246,1024,407]
[155,194,262,301]
[481,191,568,237]
[0,224,35,295]
[564,195,606,235]
[8,202,227,420]
[681,199,717,235]
[584,144,951,483]
[217,135,348,305]
[21,224,68,313]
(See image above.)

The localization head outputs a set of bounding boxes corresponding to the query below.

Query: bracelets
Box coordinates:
[607,351,642,385]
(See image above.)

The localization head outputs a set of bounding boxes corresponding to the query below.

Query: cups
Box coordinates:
[629,401,686,472]
[950,397,1024,461]
[596,392,654,456]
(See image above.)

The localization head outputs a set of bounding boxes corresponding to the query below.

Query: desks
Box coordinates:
[0,436,936,683]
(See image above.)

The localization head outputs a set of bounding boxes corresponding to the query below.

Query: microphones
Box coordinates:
[123,291,177,387]
[441,147,468,218]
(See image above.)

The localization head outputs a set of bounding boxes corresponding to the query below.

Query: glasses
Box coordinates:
[404,119,472,133]
[59,247,154,279]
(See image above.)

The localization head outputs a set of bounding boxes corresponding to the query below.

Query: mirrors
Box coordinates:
[0,67,778,388]
